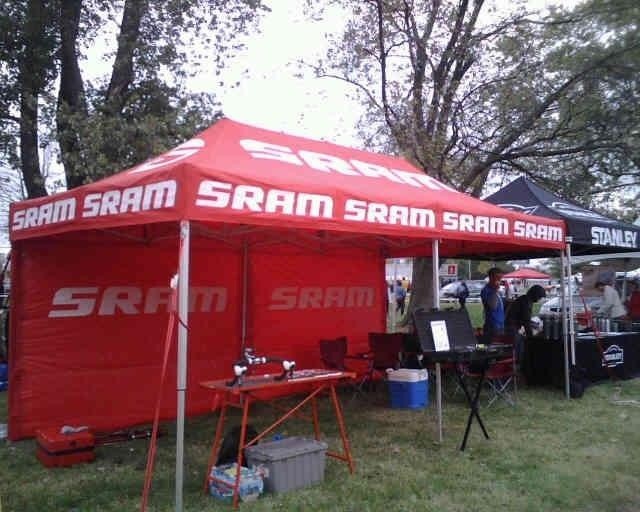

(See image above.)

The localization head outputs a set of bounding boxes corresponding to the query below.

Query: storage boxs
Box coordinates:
[387,367,431,412]
[33,425,96,469]
[241,433,331,497]
[408,305,512,361]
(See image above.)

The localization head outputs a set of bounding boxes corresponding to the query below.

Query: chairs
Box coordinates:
[367,327,412,402]
[318,333,376,410]
[462,330,522,410]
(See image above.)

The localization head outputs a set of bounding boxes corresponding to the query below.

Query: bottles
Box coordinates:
[592,316,610,332]
[237,504,238,506]
[206,463,265,505]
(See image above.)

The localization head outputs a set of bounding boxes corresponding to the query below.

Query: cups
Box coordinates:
[612,323,618,332]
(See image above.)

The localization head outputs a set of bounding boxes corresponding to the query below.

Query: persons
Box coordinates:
[480,267,507,336]
[401,276,409,297]
[456,280,469,310]
[594,281,627,320]
[504,283,546,337]
[624,279,640,319]
[394,280,407,318]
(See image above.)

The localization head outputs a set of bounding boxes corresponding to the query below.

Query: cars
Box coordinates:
[439,265,640,297]
[537,282,601,314]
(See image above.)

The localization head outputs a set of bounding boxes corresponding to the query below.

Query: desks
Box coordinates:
[198,367,360,511]
[514,328,640,398]
[415,345,505,450]
